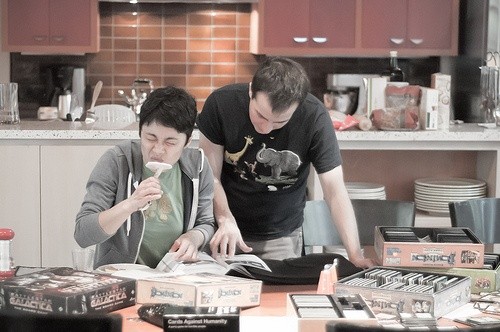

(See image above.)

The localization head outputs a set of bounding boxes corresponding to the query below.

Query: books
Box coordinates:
[96,246,272,285]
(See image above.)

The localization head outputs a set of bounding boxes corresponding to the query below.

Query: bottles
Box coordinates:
[383,50,405,83]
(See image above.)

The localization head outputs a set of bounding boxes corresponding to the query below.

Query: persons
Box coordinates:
[72,84,217,258]
[195,58,371,268]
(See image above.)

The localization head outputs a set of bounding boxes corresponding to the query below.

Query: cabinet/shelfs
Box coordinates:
[249,0,459,56]
[0,138,130,270]
[0,0,101,56]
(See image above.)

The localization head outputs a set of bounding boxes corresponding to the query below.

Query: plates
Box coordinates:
[414,176,488,215]
[95,261,148,275]
[346,181,389,200]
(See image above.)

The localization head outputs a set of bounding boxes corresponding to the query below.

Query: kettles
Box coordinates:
[116,79,155,115]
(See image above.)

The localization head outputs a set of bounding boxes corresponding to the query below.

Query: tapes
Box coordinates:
[324,264,331,270]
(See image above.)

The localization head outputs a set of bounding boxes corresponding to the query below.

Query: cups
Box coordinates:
[0,83,21,125]
[478,65,500,130]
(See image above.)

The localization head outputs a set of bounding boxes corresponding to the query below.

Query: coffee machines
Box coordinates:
[323,71,387,119]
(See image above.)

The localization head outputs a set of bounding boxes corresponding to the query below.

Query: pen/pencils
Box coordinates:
[139,177,152,206]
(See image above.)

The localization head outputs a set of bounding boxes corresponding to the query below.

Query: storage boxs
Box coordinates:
[287,292,379,332]
[331,264,472,321]
[133,272,262,310]
[400,252,500,293]
[0,267,137,314]
[374,225,486,269]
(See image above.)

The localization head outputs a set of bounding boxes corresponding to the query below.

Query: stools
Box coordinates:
[302,198,412,255]
[449,197,500,244]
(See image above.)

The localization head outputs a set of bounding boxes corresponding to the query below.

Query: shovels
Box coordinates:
[85,81,103,124]
